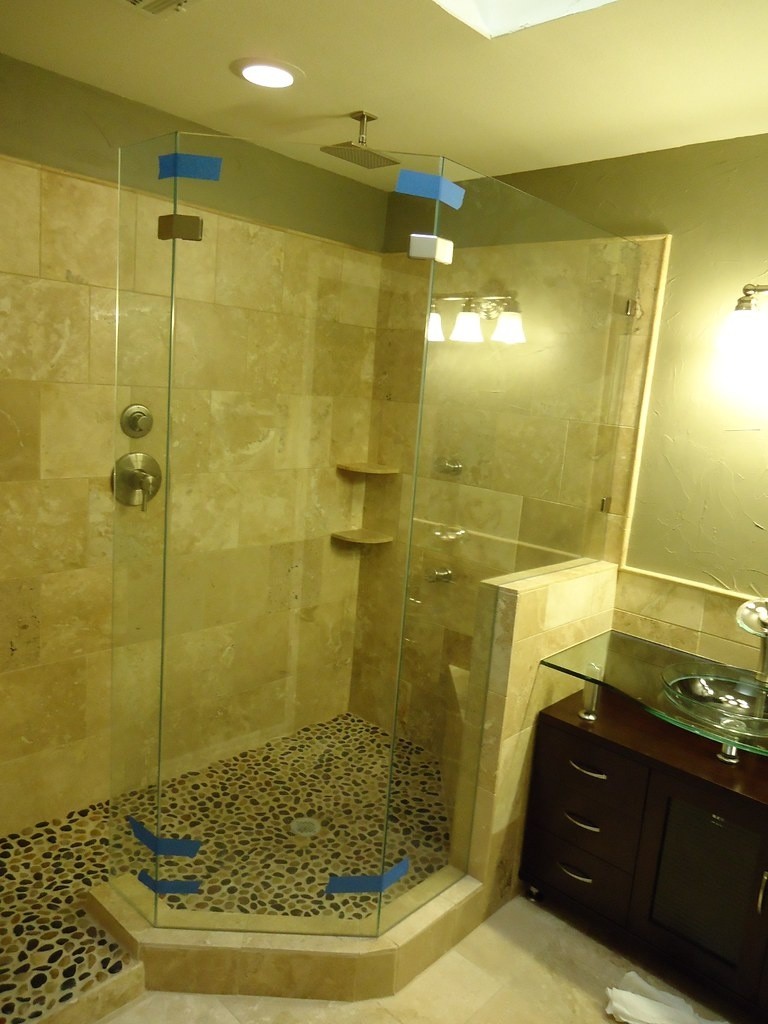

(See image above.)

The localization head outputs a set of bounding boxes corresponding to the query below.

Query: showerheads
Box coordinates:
[319,140,402,170]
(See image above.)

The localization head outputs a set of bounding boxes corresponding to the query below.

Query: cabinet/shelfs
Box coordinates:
[519,688,768,1024]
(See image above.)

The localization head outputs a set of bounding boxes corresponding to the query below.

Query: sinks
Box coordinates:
[660,661,768,738]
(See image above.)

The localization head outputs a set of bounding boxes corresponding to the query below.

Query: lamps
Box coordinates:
[427,290,524,344]
[716,284,768,347]
